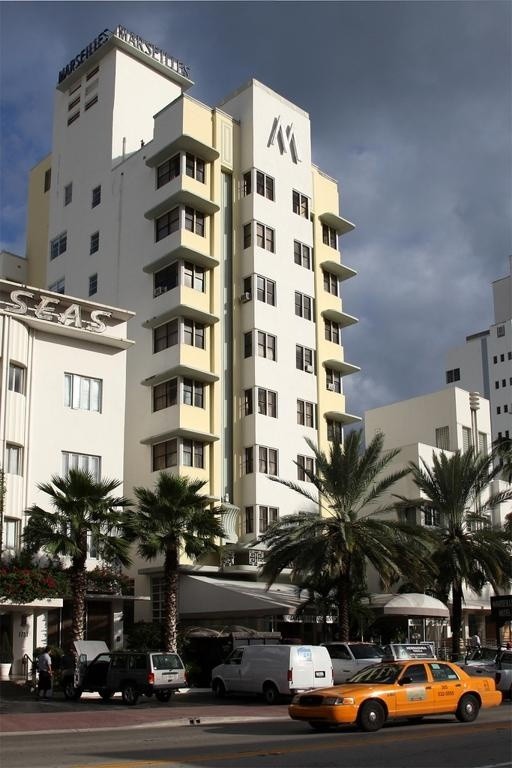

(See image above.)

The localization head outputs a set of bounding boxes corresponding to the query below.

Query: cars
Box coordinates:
[288,659,503,732]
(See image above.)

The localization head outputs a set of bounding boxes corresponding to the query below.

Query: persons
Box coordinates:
[37,646,52,697]
[470,631,482,659]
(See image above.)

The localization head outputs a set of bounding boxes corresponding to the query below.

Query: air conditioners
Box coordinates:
[152,287,162,297]
[326,382,335,391]
[305,364,314,373]
[239,292,250,302]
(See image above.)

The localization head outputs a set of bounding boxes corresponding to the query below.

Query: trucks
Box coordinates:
[212,645,335,704]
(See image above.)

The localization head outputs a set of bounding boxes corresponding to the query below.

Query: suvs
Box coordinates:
[453,646,512,694]
[62,639,186,705]
[318,641,438,684]
